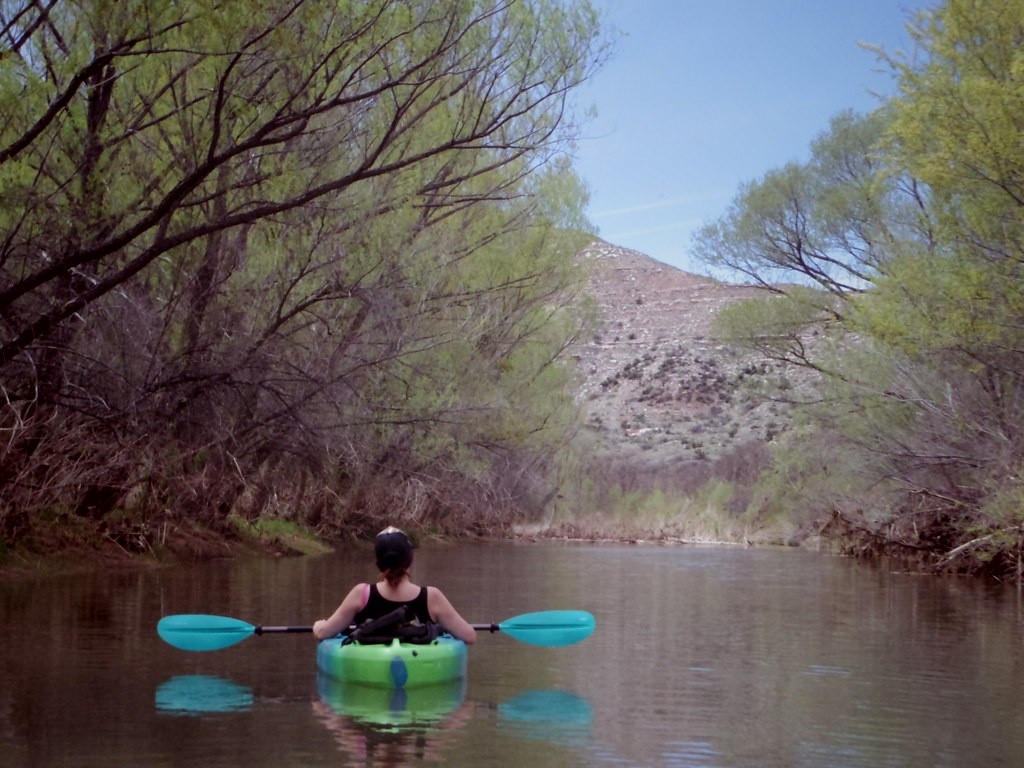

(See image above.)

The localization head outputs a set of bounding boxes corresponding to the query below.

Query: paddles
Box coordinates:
[154,608,598,653]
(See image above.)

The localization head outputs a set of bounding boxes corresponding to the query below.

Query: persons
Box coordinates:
[310,525,479,645]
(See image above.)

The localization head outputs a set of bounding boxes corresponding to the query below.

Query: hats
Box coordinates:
[372,525,414,562]
